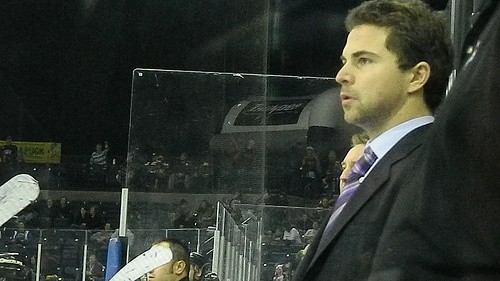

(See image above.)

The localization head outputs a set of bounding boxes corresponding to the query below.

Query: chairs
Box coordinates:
[0,158,317,281]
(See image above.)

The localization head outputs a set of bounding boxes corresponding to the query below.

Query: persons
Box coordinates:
[338,131,370,194]
[394,0,500,281]
[289,0,457,281]
[1,143,350,281]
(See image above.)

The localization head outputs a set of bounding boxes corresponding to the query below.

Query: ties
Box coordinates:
[316,145,374,245]
